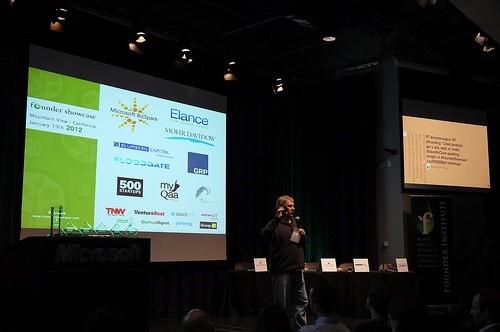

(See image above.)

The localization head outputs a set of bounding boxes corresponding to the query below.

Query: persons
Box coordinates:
[84,306,134,332]
[260,195,309,332]
[180,308,216,332]
[296,282,499,332]
[257,303,291,332]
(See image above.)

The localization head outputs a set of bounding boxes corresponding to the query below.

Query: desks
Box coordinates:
[220,270,430,320]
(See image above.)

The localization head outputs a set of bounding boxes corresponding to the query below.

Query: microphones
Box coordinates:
[295,217,301,230]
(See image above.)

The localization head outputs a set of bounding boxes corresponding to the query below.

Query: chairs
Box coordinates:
[339,263,353,272]
[304,262,320,270]
[235,262,254,270]
[379,263,394,271]
